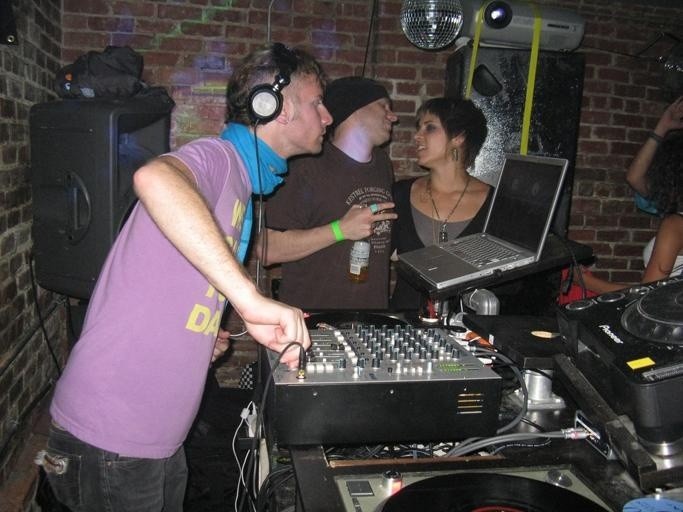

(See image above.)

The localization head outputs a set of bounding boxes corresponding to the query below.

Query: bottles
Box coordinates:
[346,206,372,281]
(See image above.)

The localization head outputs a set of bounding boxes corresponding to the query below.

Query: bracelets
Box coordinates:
[329,219,344,242]
[648,131,663,141]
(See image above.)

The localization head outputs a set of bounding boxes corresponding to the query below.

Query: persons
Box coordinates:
[624,96,682,215]
[391,97,497,312]
[558,128,683,306]
[42,42,333,511]
[253,76,398,312]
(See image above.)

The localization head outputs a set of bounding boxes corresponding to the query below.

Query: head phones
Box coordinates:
[247,53,298,124]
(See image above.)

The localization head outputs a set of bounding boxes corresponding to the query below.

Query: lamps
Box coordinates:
[400,0,463,50]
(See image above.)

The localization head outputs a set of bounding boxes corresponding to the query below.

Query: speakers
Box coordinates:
[29,97,171,300]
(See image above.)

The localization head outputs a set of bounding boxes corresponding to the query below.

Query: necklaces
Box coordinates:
[426,176,469,243]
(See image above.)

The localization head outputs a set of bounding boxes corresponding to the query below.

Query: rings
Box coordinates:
[370,203,377,213]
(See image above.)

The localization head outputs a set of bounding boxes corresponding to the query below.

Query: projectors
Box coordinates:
[463,1,586,51]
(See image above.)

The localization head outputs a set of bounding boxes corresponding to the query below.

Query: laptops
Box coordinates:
[397,151,570,292]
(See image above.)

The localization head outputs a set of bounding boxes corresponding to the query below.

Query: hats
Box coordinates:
[323,76,387,126]
